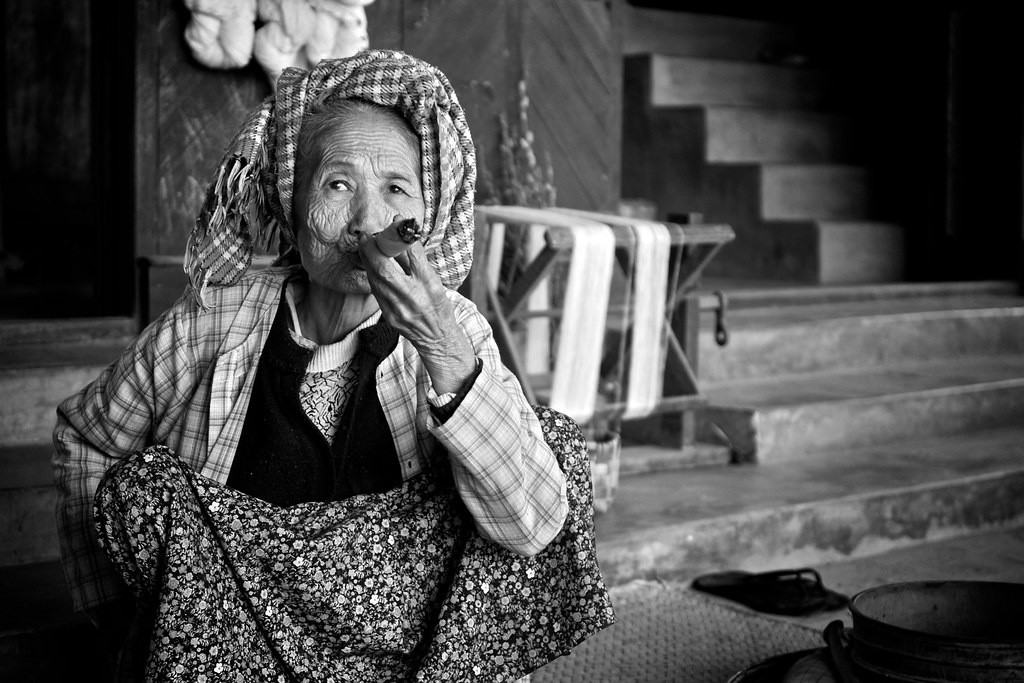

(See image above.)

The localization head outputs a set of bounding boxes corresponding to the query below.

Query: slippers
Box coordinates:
[692,566,849,619]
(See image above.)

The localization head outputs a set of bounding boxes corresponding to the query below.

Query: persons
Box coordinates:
[50,51,618,683]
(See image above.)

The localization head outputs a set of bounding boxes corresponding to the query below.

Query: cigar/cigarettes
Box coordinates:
[350,220,424,269]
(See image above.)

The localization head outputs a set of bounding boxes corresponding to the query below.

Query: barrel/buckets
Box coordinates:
[823,580,1024,683]
